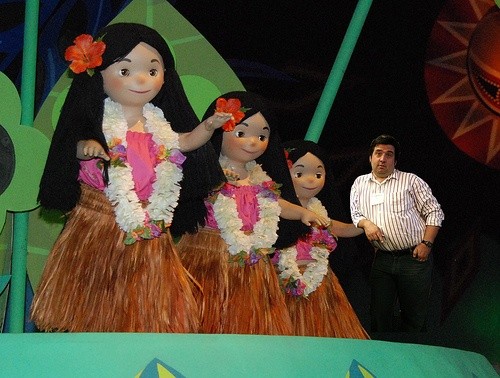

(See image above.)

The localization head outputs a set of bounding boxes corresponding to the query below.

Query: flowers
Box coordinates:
[214,98,252,132]
[64,33,106,77]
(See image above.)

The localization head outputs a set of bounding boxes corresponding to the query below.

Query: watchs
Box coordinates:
[421,239,434,248]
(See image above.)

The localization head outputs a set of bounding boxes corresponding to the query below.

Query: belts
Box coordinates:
[379,246,417,256]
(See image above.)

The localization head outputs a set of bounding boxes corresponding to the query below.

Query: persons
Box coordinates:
[30,22,235,333]
[348,135,446,344]
[172,90,331,335]
[271,140,372,339]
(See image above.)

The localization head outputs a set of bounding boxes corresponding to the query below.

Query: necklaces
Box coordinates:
[103,97,185,244]
[210,159,282,266]
[272,195,337,300]
